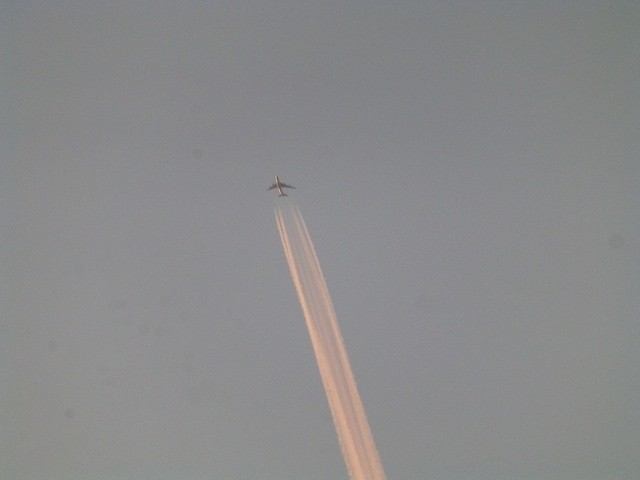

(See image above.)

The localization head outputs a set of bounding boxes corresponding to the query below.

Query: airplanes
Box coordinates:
[267,175,296,198]
